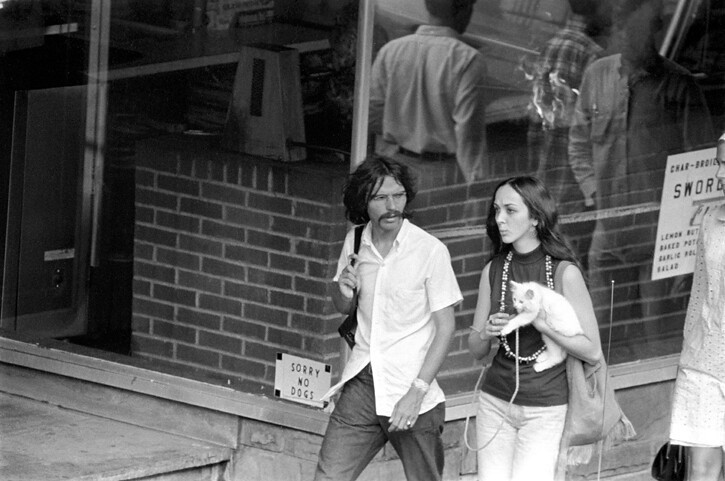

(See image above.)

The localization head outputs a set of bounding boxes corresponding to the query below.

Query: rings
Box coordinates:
[406,421,411,427]
[489,320,494,325]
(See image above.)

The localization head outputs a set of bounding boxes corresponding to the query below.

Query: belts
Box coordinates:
[399,146,456,161]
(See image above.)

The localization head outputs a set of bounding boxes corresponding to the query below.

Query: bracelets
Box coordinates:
[411,377,429,395]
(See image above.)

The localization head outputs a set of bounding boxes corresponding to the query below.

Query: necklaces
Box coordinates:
[499,251,554,362]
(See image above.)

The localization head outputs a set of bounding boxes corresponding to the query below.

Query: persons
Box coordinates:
[312,155,464,481]
[667,132,725,481]
[368,1,693,372]
[467,177,602,480]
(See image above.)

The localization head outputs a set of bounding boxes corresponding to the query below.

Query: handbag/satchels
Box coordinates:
[651,441,685,481]
[338,225,365,350]
[554,261,636,447]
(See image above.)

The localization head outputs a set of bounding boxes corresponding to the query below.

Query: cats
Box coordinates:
[498,280,583,373]
